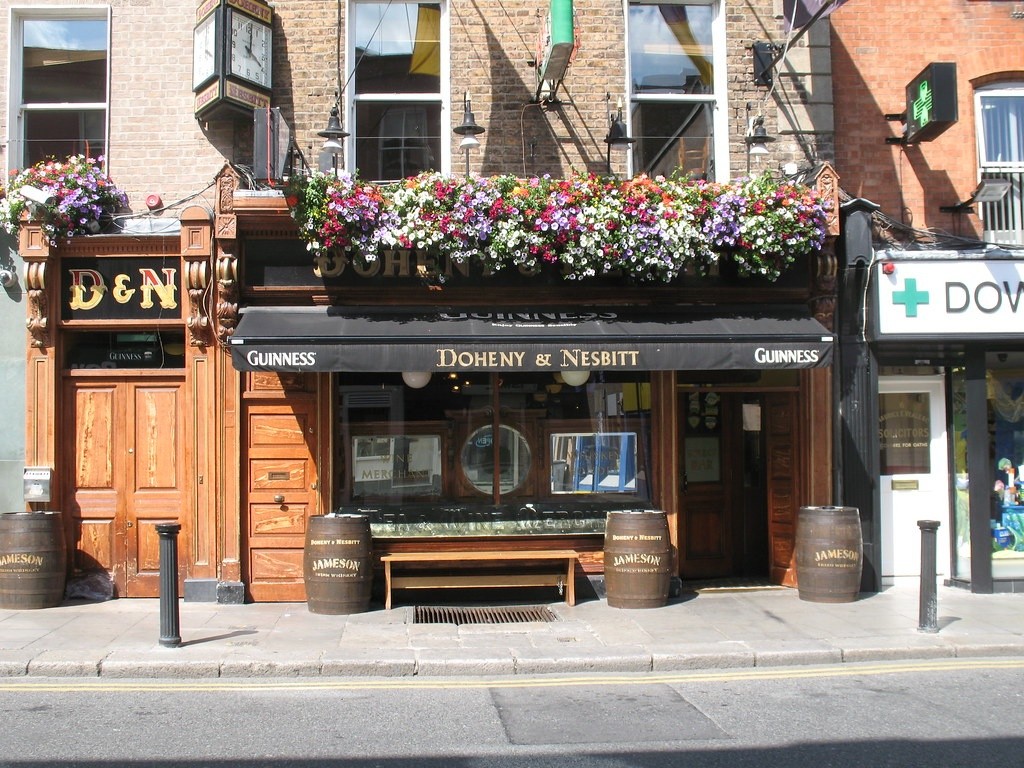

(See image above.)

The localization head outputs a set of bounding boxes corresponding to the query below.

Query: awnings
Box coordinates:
[228,303,833,373]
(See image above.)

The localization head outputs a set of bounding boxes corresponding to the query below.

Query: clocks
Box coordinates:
[226,6,274,97]
[191,3,221,93]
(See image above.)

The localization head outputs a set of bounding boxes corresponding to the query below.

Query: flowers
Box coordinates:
[275,164,840,290]
[1,152,129,239]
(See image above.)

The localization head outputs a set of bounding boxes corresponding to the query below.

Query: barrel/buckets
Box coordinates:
[795,506,863,604]
[0,512,69,610]
[603,509,672,609]
[304,514,375,616]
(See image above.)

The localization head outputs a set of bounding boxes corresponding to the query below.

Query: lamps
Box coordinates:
[316,88,350,178]
[602,96,637,174]
[454,91,487,179]
[744,100,775,175]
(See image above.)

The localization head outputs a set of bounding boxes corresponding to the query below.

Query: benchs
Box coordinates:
[382,550,581,610]
